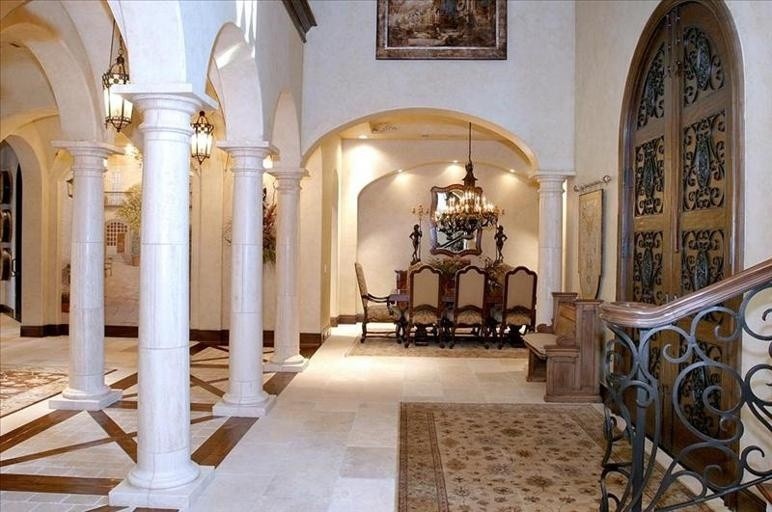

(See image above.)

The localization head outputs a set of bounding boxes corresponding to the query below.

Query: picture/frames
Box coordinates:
[375,0,509,62]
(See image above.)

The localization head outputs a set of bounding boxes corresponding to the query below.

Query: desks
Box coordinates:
[386,293,537,344]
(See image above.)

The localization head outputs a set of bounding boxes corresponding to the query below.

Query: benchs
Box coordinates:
[353,261,401,344]
[518,291,603,404]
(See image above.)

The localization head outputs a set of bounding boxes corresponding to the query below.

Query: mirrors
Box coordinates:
[430,183,484,259]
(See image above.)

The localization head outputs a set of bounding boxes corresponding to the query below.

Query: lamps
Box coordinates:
[100,34,140,133]
[190,108,214,166]
[438,121,504,242]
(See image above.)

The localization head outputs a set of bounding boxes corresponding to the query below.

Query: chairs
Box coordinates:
[393,261,538,350]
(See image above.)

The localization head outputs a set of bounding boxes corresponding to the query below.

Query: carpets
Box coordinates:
[399,400,715,512]
[0,360,117,419]
[344,332,530,361]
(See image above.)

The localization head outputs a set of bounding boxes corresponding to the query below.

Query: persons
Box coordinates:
[409,224,422,263]
[494,225,507,261]
